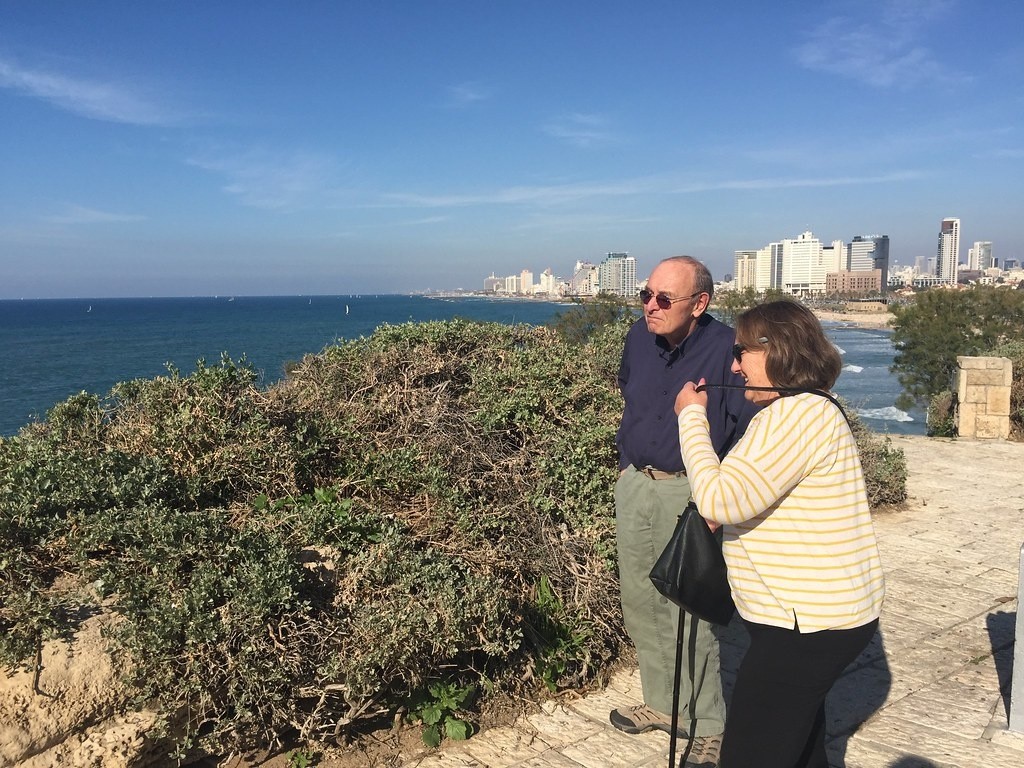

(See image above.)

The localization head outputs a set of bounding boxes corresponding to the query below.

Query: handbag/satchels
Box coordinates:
[648,501,737,627]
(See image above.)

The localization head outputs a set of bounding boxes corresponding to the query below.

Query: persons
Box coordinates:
[610,255,767,767]
[674,300,885,768]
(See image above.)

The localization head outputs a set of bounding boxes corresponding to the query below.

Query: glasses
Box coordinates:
[640,290,705,310]
[732,337,769,363]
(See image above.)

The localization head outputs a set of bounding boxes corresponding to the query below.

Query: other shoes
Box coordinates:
[680,730,724,768]
[609,703,690,739]
